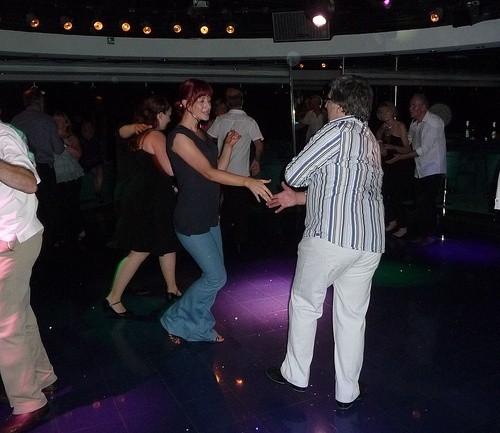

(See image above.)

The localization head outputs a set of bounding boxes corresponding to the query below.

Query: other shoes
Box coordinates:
[386,225,398,234]
[388,232,408,239]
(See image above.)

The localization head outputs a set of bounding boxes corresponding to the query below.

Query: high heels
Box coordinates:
[166,291,183,299]
[103,299,134,318]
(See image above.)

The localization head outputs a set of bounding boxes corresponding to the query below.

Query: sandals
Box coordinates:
[215,336,225,343]
[168,334,182,346]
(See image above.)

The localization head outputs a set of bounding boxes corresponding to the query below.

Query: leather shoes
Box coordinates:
[337,382,366,409]
[265,367,305,393]
[0,402,49,433]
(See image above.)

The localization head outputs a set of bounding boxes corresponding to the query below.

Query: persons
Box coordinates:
[102,94,184,320]
[375,100,412,239]
[159,78,273,347]
[293,91,328,144]
[266,74,386,415]
[0,117,59,433]
[11,85,105,241]
[385,93,447,247]
[199,88,265,244]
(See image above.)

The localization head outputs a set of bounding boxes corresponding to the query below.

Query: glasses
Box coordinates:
[323,96,332,102]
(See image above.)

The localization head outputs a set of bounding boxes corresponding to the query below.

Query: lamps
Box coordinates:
[226,21,237,33]
[304,0,329,30]
[199,22,210,35]
[171,21,182,33]
[59,16,73,30]
[92,16,104,31]
[429,8,445,24]
[25,13,40,28]
[139,20,152,35]
[119,18,131,32]
[381,0,393,10]
